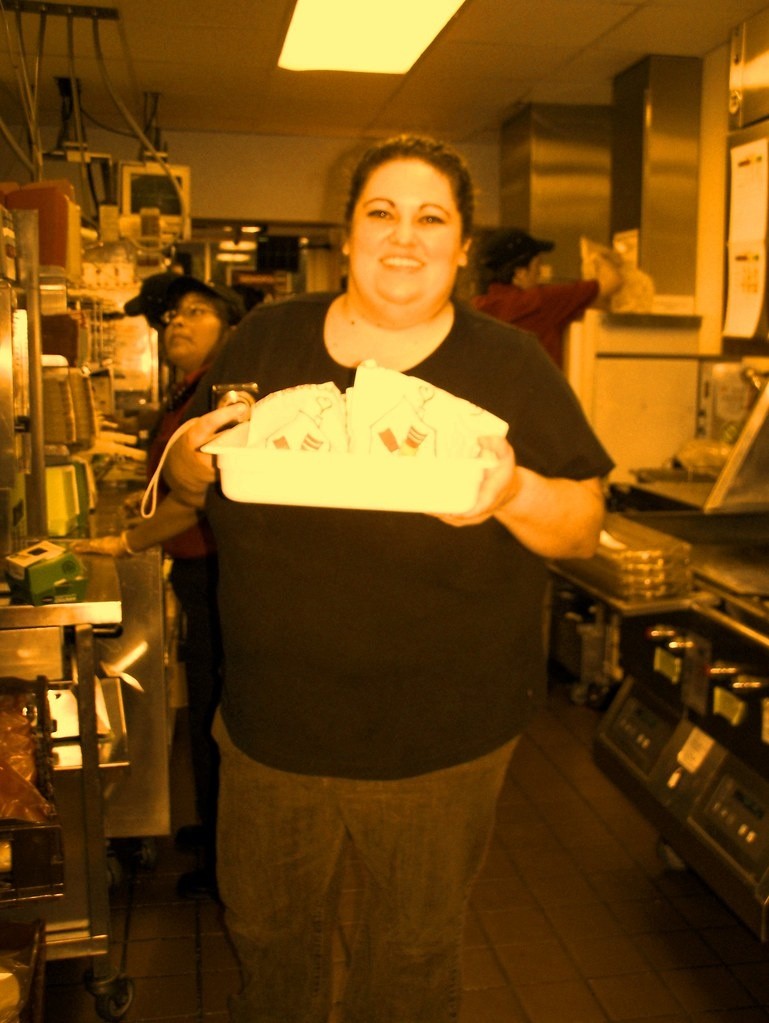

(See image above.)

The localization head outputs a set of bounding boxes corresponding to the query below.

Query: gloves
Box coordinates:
[75,531,142,559]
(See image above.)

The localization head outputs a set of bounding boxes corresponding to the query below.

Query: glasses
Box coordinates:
[159,306,212,321]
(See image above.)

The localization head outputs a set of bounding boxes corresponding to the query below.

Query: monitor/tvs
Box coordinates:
[121,166,184,223]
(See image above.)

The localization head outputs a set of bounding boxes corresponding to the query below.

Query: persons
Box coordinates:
[101,272,184,435]
[471,229,623,373]
[74,282,246,853]
[161,136,617,1023]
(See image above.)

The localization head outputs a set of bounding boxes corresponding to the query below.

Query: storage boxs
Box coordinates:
[200,422,497,513]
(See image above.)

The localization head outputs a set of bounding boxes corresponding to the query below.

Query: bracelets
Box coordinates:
[121,532,141,555]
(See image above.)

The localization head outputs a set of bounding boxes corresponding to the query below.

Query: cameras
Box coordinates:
[210,383,259,434]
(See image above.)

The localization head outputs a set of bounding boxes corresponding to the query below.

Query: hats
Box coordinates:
[473,225,556,269]
[141,274,245,327]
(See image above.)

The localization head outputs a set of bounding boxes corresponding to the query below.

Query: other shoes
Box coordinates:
[176,824,216,898]
